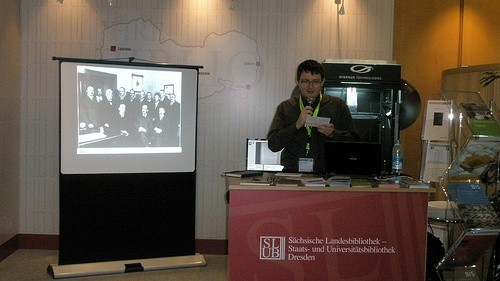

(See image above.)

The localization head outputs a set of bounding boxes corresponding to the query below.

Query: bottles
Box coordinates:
[392,140,403,181]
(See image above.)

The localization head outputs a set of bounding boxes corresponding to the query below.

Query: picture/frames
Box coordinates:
[421,99,454,142]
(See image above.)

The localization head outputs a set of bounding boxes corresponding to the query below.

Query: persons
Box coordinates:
[79,86,180,147]
[267,59,354,174]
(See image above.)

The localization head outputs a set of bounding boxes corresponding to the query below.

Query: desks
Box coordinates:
[224,174,436,281]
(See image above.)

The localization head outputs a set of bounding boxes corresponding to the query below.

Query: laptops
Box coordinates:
[245,138,284,173]
[323,141,383,178]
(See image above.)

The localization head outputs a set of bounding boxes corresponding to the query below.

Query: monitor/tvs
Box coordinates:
[324,86,382,116]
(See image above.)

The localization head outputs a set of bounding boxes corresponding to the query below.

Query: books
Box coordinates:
[437,101,500,281]
[225,171,429,188]
